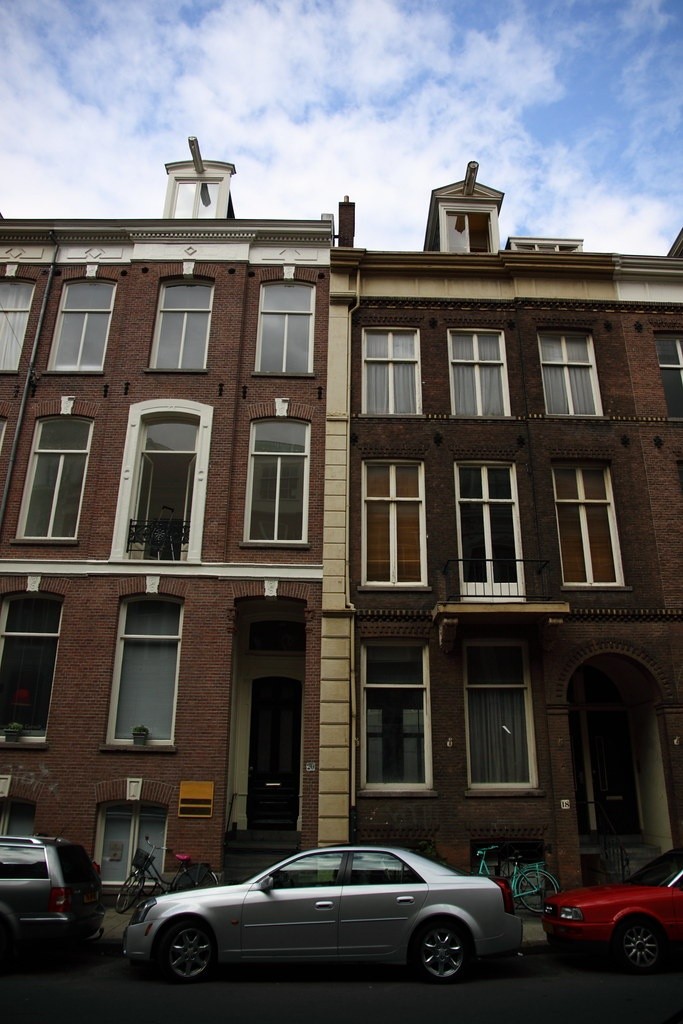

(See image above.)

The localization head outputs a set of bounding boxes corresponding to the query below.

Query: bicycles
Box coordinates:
[475,846,561,913]
[115,837,218,915]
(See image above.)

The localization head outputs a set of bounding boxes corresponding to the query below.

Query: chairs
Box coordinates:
[129,505,176,561]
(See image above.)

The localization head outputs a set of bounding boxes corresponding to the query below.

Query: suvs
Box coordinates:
[0,836,106,977]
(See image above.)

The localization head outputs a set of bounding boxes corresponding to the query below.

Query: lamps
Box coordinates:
[673,733,683,745]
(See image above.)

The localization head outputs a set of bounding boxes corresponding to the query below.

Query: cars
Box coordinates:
[120,846,523,984]
[542,848,683,971]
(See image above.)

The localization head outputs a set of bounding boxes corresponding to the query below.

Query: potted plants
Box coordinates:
[3,723,23,742]
[131,725,149,746]
